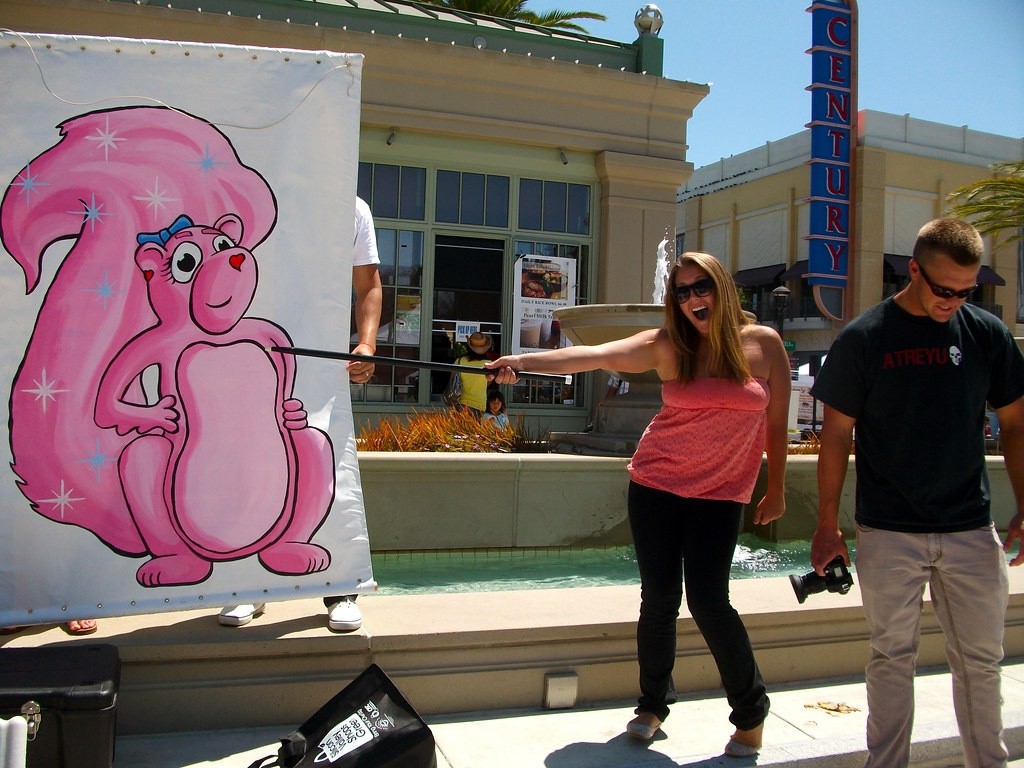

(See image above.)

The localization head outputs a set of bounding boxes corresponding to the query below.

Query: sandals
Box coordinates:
[66,619,97,635]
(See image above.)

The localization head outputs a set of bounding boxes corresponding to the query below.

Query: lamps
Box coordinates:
[387,127,396,145]
[560,150,568,165]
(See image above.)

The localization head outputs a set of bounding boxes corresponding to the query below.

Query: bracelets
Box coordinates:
[360,342,375,352]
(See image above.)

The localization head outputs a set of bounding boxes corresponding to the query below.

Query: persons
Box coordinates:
[444,333,509,433]
[810,218,1024,768]
[218,196,383,630]
[480,252,791,758]
[0,618,97,635]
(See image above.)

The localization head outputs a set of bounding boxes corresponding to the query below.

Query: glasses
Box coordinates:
[671,278,714,305]
[917,261,978,299]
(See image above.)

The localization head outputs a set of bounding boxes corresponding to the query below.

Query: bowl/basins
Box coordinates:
[528,263,560,277]
[521,278,554,298]
[543,273,568,291]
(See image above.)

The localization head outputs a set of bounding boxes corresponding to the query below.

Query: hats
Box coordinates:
[468,332,491,354]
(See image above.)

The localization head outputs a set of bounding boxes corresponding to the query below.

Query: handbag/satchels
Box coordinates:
[248,663,437,768]
[443,358,463,405]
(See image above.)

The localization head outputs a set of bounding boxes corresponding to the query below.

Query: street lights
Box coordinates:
[771,283,790,341]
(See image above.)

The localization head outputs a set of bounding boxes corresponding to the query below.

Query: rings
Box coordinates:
[366,370,369,377]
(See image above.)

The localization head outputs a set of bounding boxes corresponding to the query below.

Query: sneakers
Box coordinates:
[219,602,266,626]
[328,595,363,630]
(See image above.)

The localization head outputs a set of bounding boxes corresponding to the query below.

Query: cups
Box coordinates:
[521,320,541,348]
[540,320,552,342]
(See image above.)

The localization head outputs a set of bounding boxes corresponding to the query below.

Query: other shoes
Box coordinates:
[725,720,764,758]
[627,711,662,741]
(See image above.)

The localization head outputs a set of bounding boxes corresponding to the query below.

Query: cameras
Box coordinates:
[788,556,854,604]
